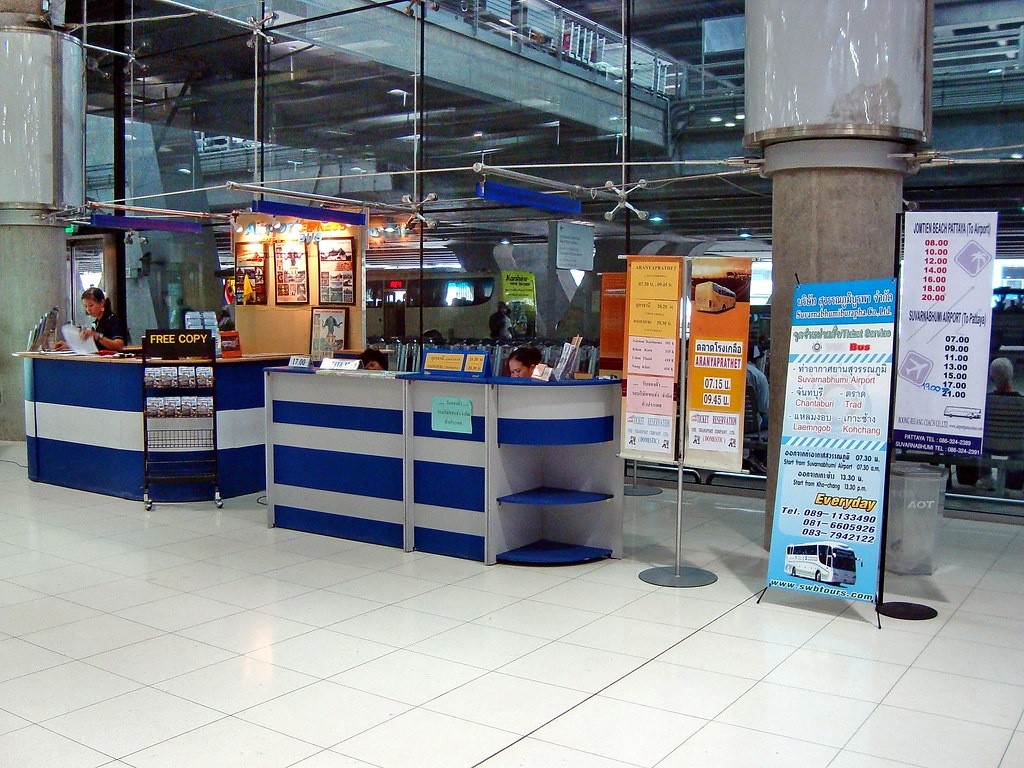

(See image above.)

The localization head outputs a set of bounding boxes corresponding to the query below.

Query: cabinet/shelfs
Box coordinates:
[497,487,615,567]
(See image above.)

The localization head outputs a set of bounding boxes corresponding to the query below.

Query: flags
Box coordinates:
[221,280,236,306]
[243,275,252,306]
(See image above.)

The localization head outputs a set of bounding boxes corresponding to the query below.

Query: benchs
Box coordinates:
[744,387,769,475]
[895,396,1024,494]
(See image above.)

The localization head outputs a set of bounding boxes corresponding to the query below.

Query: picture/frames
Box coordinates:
[273,238,310,304]
[235,241,268,306]
[310,307,350,361]
[317,235,356,306]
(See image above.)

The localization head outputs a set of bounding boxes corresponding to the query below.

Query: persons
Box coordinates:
[56,287,129,352]
[216,309,235,331]
[358,348,388,370]
[172,297,195,330]
[1006,300,1017,312]
[507,347,542,378]
[976,358,1024,490]
[488,301,528,340]
[678,338,770,476]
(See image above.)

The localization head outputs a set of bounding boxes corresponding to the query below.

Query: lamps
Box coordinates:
[272,215,287,232]
[230,214,243,233]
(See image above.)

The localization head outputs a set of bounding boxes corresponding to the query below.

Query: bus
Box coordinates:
[365,264,539,340]
[694,281,736,312]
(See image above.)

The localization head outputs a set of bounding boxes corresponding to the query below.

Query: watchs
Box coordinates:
[96,332,103,342]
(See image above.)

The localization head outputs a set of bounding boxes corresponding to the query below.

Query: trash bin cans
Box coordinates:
[886,460,949,576]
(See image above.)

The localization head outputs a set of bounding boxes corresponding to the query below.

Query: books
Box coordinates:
[145,366,213,418]
